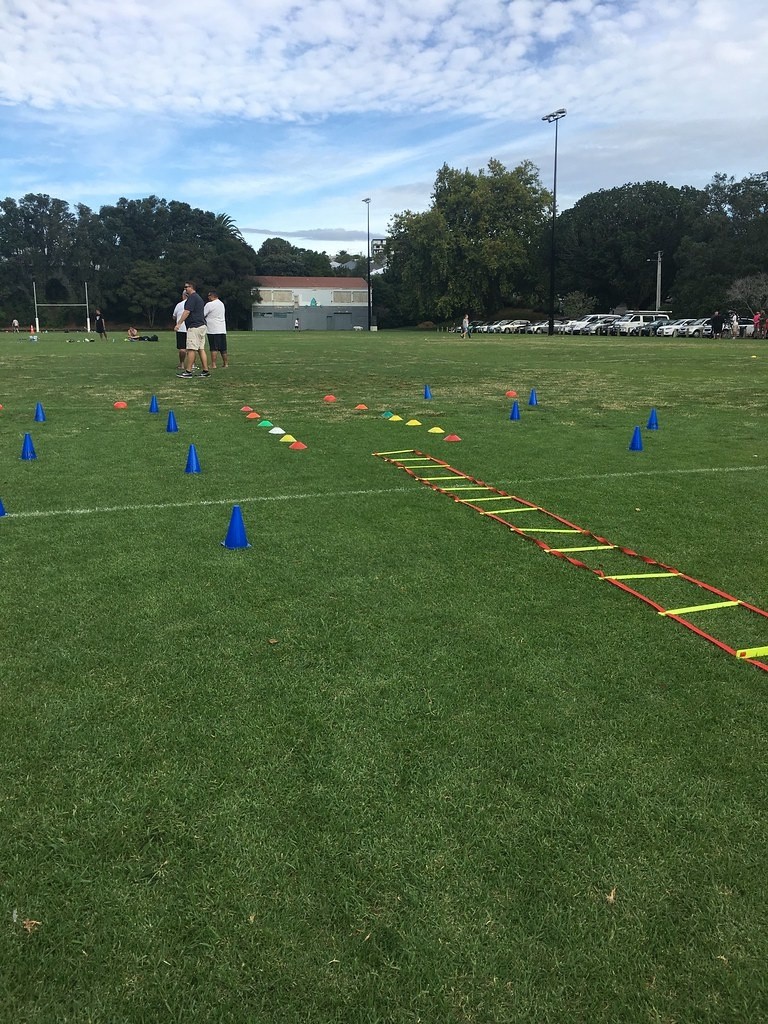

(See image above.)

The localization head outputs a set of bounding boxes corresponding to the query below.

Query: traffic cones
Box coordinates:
[510,400,521,420]
[30,325,35,335]
[185,443,202,473]
[647,409,659,431]
[148,395,159,413]
[529,389,537,406]
[34,401,47,422]
[167,411,178,432]
[222,506,255,553]
[0,500,7,517]
[20,433,37,462]
[424,385,432,399]
[629,425,645,452]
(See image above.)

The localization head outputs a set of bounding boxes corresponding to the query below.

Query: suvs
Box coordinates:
[572,314,621,336]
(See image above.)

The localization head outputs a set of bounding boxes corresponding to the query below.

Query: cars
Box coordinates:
[723,318,764,338]
[456,320,577,335]
[635,317,714,338]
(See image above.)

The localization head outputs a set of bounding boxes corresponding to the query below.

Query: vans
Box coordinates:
[613,310,669,336]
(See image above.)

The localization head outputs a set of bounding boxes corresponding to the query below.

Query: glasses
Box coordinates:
[207,294,212,297]
[184,286,191,289]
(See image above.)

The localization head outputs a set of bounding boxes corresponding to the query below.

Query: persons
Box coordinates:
[174,281,212,379]
[462,318,471,338]
[711,311,719,339]
[731,312,738,339]
[752,312,761,338]
[712,313,725,340]
[460,314,469,338]
[293,318,300,332]
[758,311,767,339]
[172,289,198,369]
[94,309,108,341]
[12,318,19,333]
[204,290,229,368]
[127,326,140,339]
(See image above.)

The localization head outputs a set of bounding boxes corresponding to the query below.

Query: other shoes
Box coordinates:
[177,365,184,369]
[192,364,198,370]
[460,334,465,339]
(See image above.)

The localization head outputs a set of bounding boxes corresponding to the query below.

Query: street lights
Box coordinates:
[362,198,372,332]
[542,109,568,336]
[647,258,662,311]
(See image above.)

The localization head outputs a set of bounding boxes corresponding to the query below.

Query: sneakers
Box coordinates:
[198,370,211,378]
[177,371,193,378]
